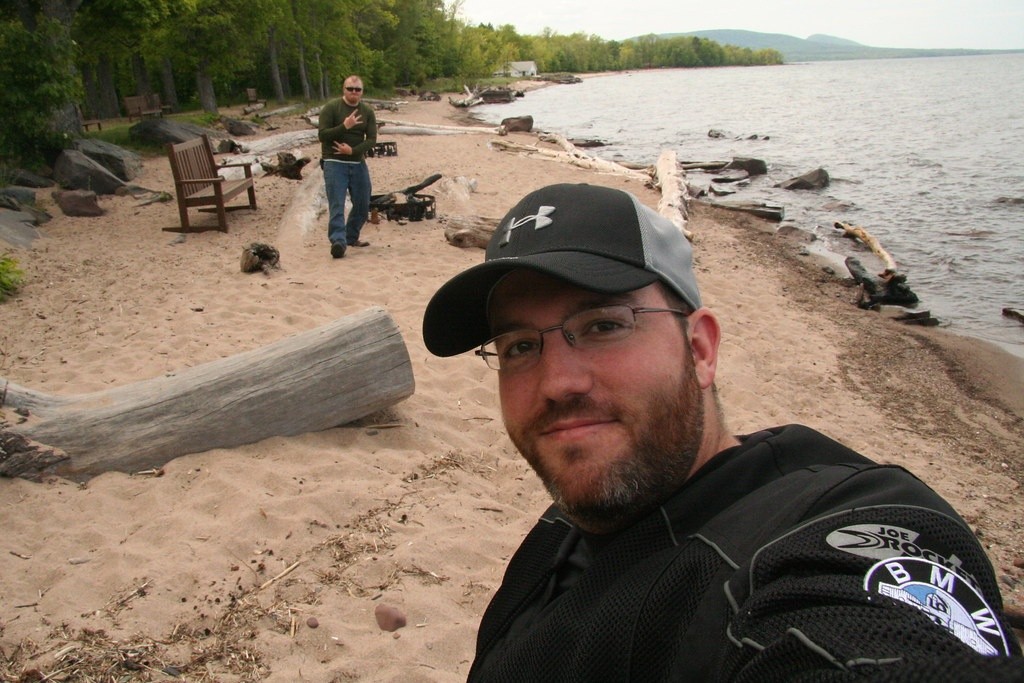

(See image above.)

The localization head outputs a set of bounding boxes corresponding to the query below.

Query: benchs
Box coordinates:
[126,96,163,123]
[75,104,102,132]
[247,88,268,107]
[167,134,257,234]
[152,94,173,114]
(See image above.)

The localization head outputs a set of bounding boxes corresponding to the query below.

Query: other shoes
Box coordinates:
[331,243,346,258]
[345,239,371,247]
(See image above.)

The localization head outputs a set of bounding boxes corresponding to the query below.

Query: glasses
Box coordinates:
[345,86,362,92]
[476,302,689,368]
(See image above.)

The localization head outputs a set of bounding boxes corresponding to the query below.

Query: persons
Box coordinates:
[422,185,1023,683]
[318,75,378,257]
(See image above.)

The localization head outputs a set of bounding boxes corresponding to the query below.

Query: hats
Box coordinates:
[422,184,706,357]
